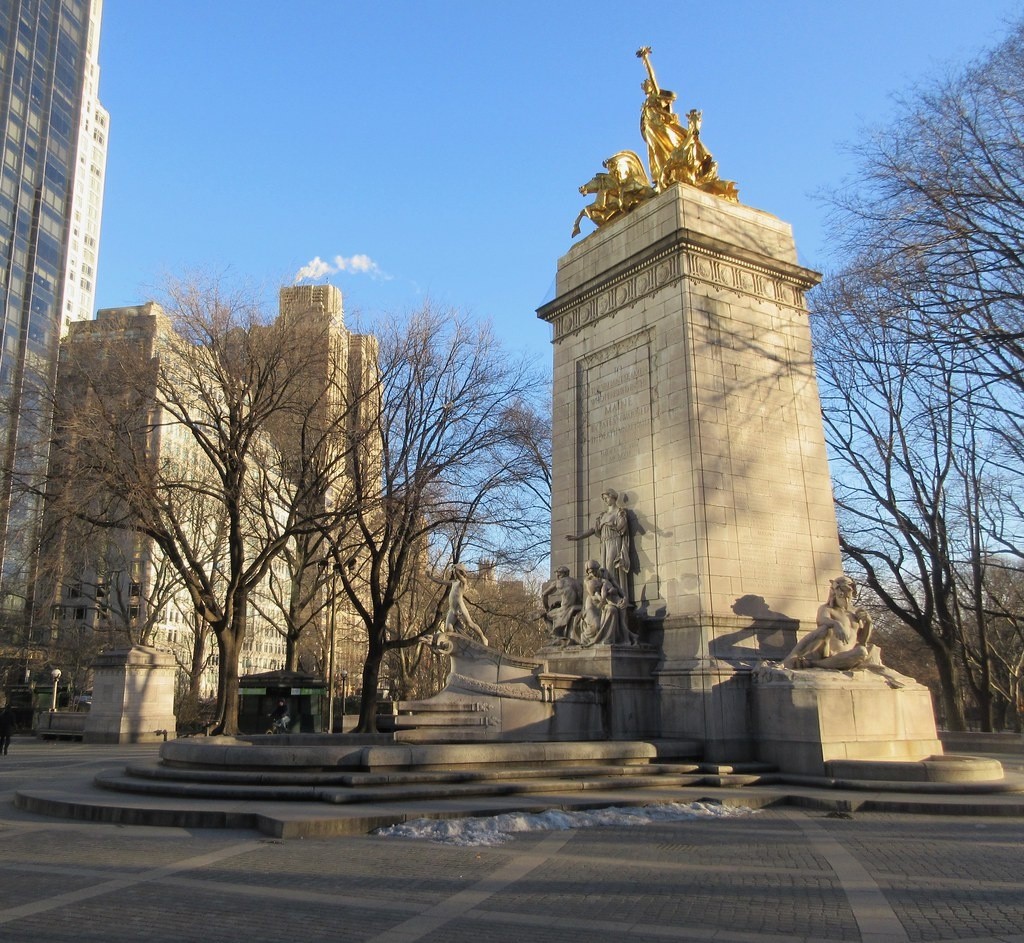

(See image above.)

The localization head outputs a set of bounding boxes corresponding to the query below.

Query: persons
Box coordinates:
[780,577,873,669]
[580,559,639,647]
[565,488,631,601]
[541,566,582,645]
[0,704,18,756]
[270,699,291,731]
[573,48,711,237]
[426,564,488,647]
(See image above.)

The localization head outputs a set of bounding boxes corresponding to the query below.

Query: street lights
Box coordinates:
[49,669,61,713]
[340,670,349,715]
[319,557,357,734]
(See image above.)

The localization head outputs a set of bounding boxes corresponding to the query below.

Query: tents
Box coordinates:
[212,669,326,733]
